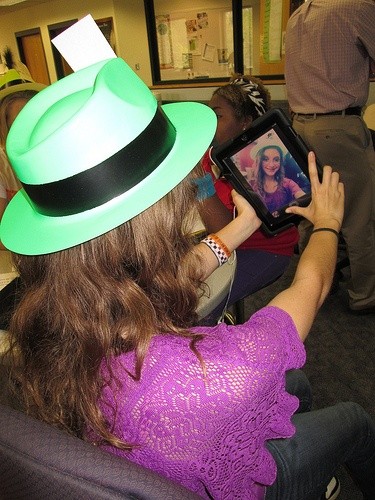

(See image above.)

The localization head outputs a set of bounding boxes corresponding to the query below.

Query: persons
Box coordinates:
[282,1,375,310]
[176,71,300,326]
[240,138,312,216]
[0,56,375,500]
[0,68,49,251]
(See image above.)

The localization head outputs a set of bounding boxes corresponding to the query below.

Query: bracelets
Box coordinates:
[310,228,339,238]
[201,233,231,267]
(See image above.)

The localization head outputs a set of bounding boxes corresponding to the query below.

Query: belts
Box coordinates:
[291,105,362,118]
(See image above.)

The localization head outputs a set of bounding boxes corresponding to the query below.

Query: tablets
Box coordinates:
[214,107,323,238]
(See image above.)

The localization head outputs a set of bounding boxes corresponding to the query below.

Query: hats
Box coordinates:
[0,68,50,101]
[249,140,286,161]
[0,58,219,256]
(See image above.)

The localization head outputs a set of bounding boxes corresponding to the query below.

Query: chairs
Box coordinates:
[0,403,341,500]
